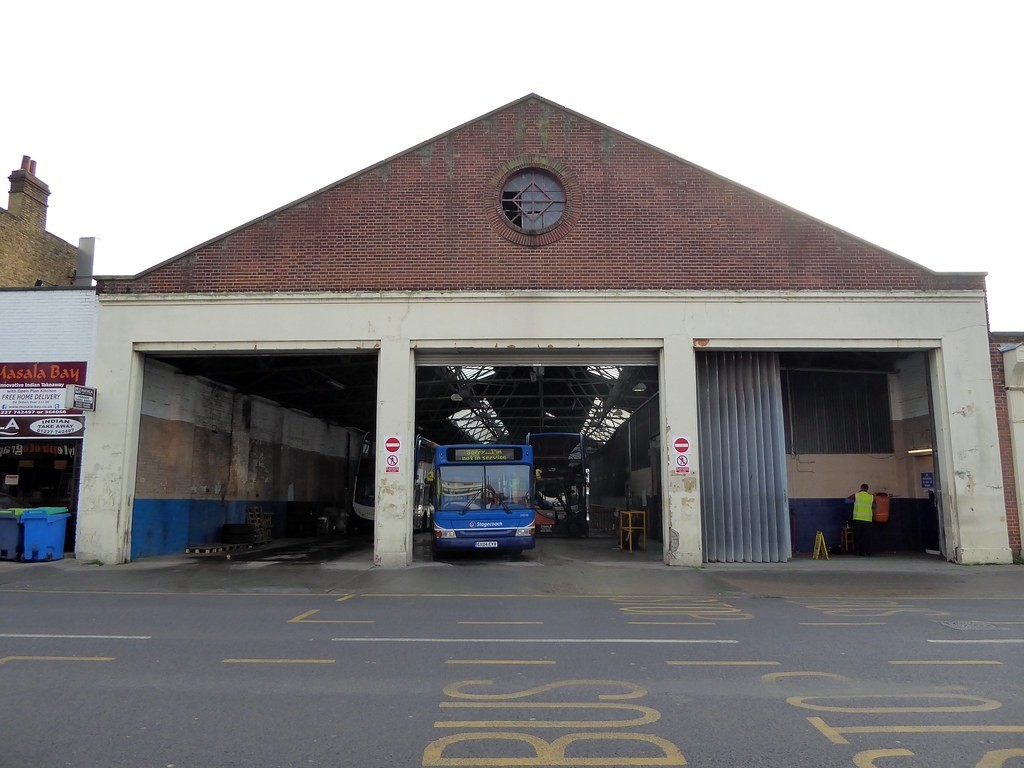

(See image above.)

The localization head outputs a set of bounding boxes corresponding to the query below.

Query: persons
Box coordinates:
[845,484,877,557]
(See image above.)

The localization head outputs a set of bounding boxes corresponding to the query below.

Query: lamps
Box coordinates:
[473,414,481,421]
[451,392,463,401]
[614,408,623,416]
[632,383,647,391]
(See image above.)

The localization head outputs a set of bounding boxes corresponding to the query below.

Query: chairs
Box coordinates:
[444,494,468,510]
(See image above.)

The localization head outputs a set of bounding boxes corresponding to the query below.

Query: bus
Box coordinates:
[353,432,441,533]
[526,430,590,539]
[426,441,542,552]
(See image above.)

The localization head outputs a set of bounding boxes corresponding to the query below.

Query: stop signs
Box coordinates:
[385,437,401,453]
[674,438,689,454]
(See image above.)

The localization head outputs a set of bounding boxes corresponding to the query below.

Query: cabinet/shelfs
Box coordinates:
[288,501,319,537]
